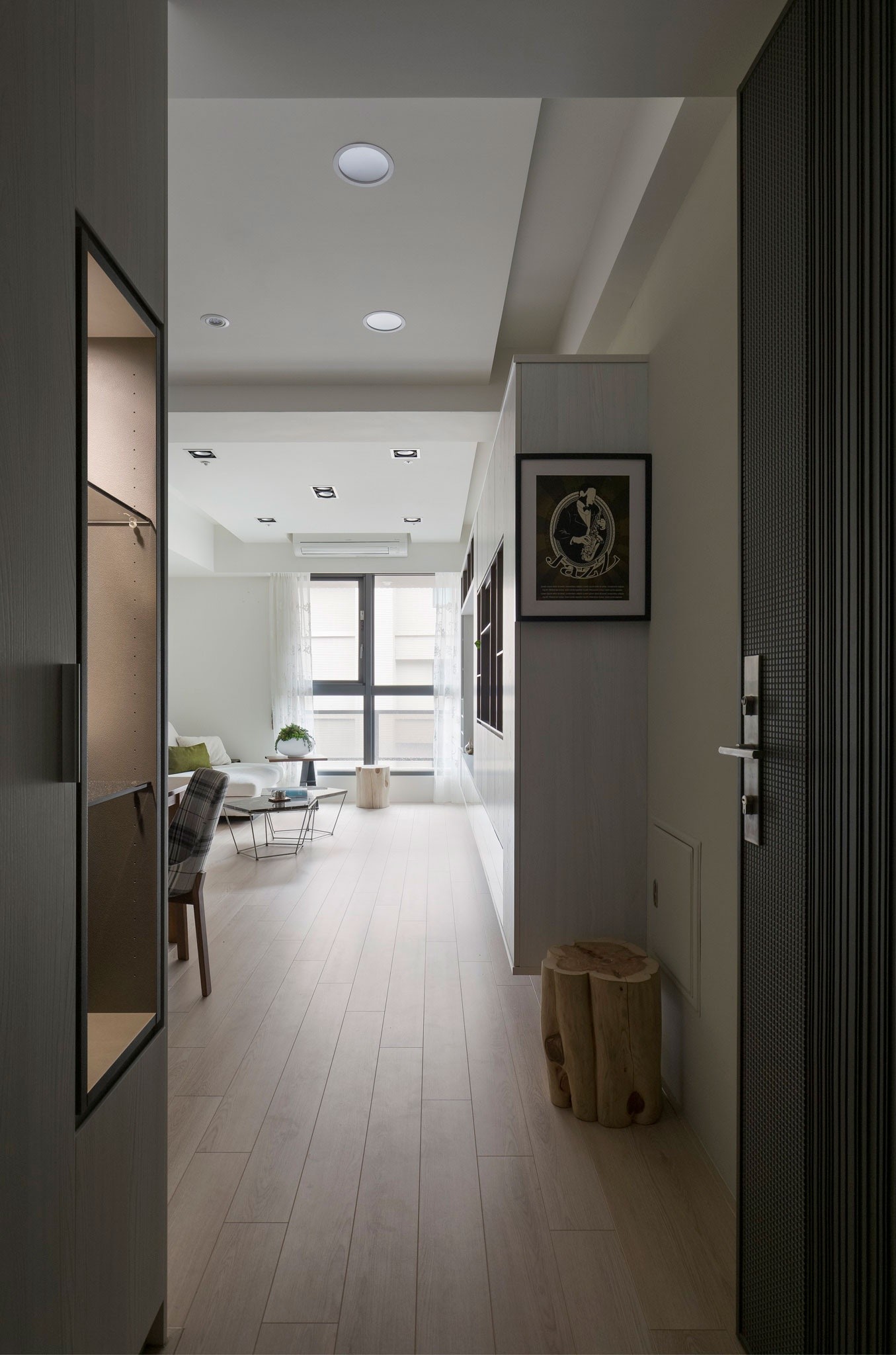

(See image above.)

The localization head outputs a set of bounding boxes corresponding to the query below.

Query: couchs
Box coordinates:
[167,722,285,820]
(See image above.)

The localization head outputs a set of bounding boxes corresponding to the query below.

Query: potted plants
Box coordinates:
[274,722,316,758]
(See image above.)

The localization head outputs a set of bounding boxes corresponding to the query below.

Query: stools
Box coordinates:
[540,937,663,1127]
[356,765,391,808]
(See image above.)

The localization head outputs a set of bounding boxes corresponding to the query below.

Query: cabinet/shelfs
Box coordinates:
[1,0,168,1355]
[458,354,650,975]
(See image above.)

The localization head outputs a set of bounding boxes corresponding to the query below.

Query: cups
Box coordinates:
[272,790,286,799]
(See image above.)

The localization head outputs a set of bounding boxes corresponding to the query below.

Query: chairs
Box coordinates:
[168,768,230,997]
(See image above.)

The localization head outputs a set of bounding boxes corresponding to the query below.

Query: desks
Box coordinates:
[267,756,328,810]
[167,776,193,961]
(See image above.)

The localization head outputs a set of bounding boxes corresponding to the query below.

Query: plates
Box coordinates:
[268,796,291,802]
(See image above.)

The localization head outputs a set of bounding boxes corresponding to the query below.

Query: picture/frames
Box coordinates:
[514,452,651,621]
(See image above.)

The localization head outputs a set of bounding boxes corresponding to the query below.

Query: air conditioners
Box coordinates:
[293,533,409,559]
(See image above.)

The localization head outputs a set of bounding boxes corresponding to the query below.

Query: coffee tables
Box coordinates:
[222,797,317,861]
[260,785,348,841]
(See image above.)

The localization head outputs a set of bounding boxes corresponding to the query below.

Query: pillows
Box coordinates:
[174,734,231,765]
[168,742,213,775]
